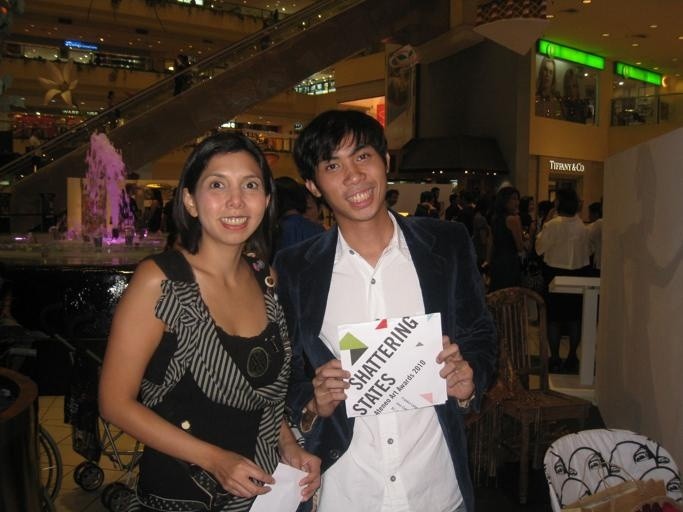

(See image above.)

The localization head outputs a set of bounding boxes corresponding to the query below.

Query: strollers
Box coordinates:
[54,284,148,512]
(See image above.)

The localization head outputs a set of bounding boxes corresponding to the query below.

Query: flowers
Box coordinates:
[38,58,99,134]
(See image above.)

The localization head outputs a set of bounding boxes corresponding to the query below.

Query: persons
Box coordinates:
[29,127,43,172]
[144,188,163,233]
[183,55,191,92]
[385,187,399,213]
[536,56,566,120]
[173,54,184,96]
[273,110,504,512]
[97,130,322,512]
[559,67,594,124]
[120,182,139,226]
[298,183,330,232]
[271,177,317,228]
[263,8,279,48]
[108,90,117,130]
[160,187,180,234]
[415,181,603,369]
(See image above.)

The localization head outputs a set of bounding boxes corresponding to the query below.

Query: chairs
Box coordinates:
[0,324,106,423]
[480,287,592,504]
[544,427,683,512]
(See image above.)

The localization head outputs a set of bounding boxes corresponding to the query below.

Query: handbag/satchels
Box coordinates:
[563,453,665,512]
